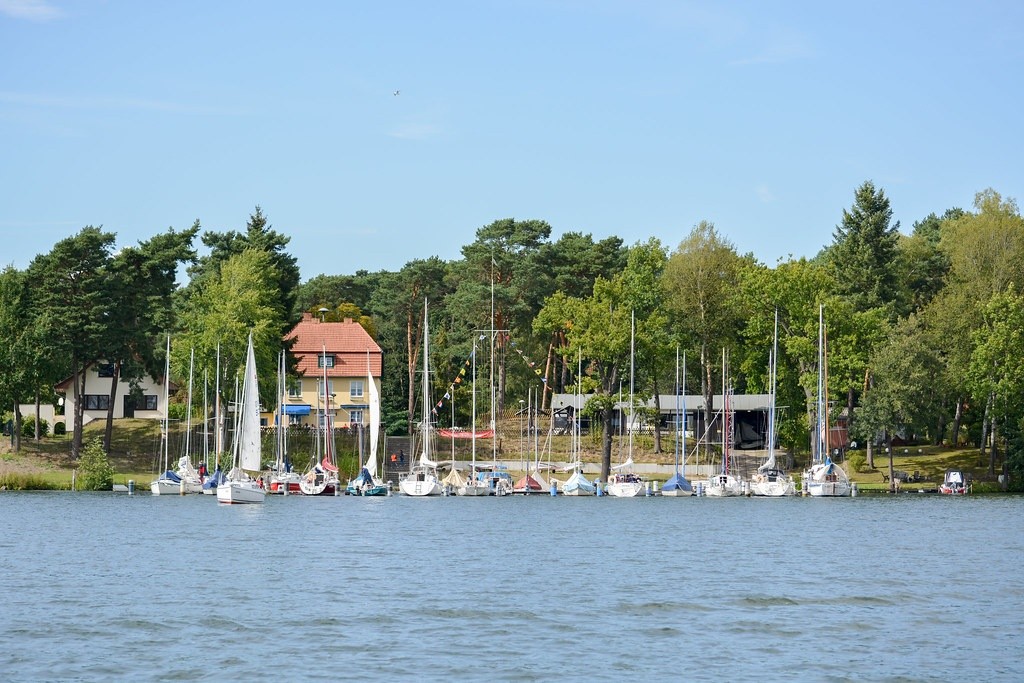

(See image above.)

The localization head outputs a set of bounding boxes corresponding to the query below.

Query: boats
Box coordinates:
[937,469,966,496]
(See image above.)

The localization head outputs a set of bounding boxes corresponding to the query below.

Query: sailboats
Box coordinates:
[151,294,855,500]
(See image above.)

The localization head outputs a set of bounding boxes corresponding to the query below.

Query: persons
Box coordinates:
[256,477,263,490]
[311,423,316,430]
[477,467,482,472]
[304,423,310,430]
[626,474,642,483]
[849,438,858,449]
[343,423,349,429]
[825,453,834,482]
[400,449,406,468]
[361,465,376,489]
[949,481,961,493]
[198,460,206,483]
[389,451,399,469]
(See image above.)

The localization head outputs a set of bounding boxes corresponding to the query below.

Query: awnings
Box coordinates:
[438,428,495,439]
[281,405,311,415]
